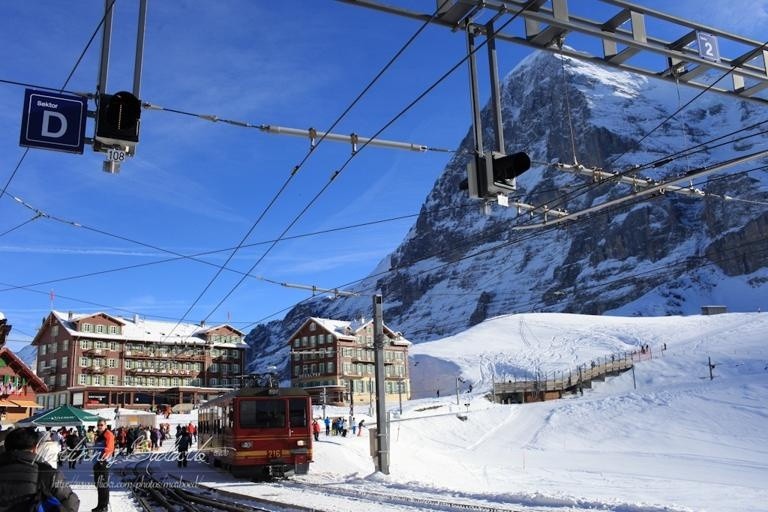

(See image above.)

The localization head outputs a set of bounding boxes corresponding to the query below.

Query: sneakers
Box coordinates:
[92,507,108,512]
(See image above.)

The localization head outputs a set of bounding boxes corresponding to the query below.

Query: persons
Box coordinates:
[0,420,200,468]
[113,406,120,419]
[90,419,114,512]
[0,428,81,511]
[150,403,172,418]
[312,413,365,440]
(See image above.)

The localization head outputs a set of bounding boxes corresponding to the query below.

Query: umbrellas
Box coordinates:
[15,403,110,427]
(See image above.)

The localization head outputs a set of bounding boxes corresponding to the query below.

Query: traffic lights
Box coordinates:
[94,88,141,148]
[483,149,531,198]
[457,161,475,201]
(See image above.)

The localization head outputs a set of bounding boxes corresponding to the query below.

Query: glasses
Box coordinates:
[97,424,103,426]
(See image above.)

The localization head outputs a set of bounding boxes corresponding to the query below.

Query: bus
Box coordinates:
[196,384,316,479]
